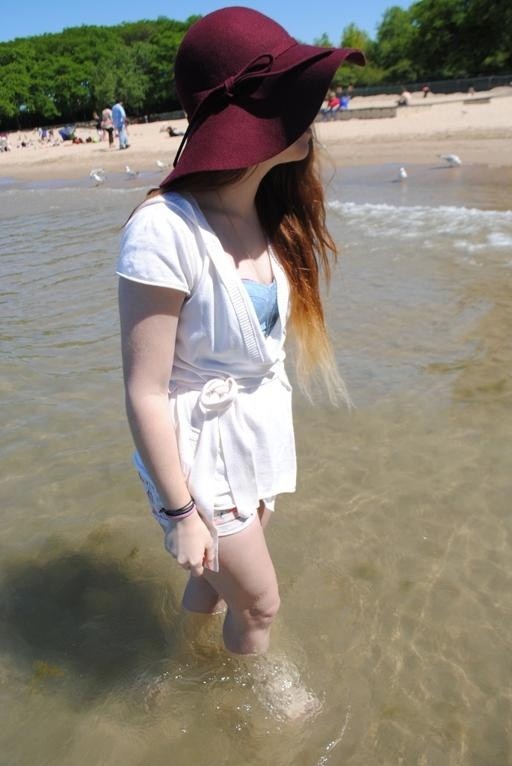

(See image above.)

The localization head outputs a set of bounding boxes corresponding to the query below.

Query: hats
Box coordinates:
[159,7,366,187]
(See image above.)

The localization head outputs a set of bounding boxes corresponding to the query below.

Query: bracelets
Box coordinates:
[157,496,196,521]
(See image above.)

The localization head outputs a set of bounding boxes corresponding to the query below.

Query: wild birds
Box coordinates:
[90,171,106,186]
[155,159,171,171]
[397,166,407,183]
[123,165,141,180]
[437,153,462,169]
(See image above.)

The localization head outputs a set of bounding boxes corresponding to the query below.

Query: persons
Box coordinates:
[1,97,131,155]
[335,91,352,113]
[396,88,412,105]
[160,126,184,136]
[111,7,369,723]
[320,92,340,121]
